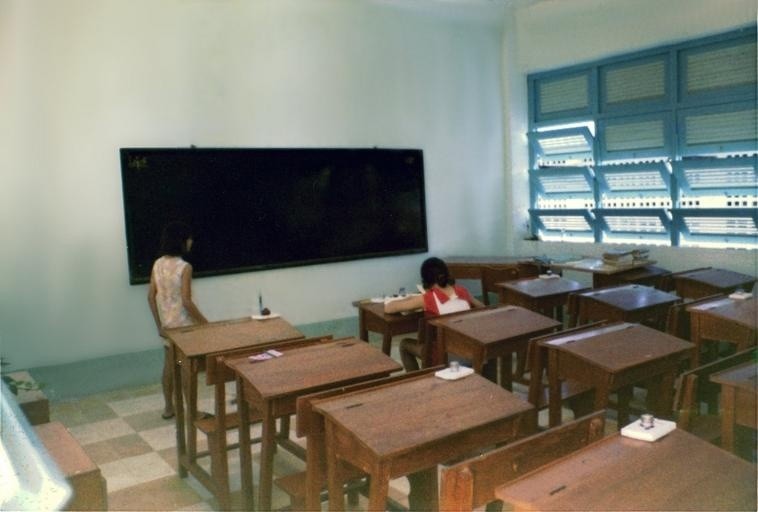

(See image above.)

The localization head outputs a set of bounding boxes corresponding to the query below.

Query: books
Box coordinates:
[251,313,280,320]
[538,273,560,279]
[600,249,650,267]
[620,417,676,442]
[435,365,475,381]
[729,292,753,300]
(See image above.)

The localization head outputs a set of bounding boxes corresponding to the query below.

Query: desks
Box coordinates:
[4,370,50,426]
[33,420,110,511]
[164,255,758,511]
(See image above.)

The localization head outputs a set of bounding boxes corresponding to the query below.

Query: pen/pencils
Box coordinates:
[262,349,278,359]
[259,295,262,315]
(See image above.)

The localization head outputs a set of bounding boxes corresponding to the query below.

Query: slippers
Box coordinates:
[162,413,174,419]
[202,412,214,419]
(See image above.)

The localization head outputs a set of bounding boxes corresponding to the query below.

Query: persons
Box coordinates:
[384,257,488,372]
[147,227,215,421]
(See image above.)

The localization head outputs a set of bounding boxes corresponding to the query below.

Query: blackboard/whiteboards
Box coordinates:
[120,147,429,285]
